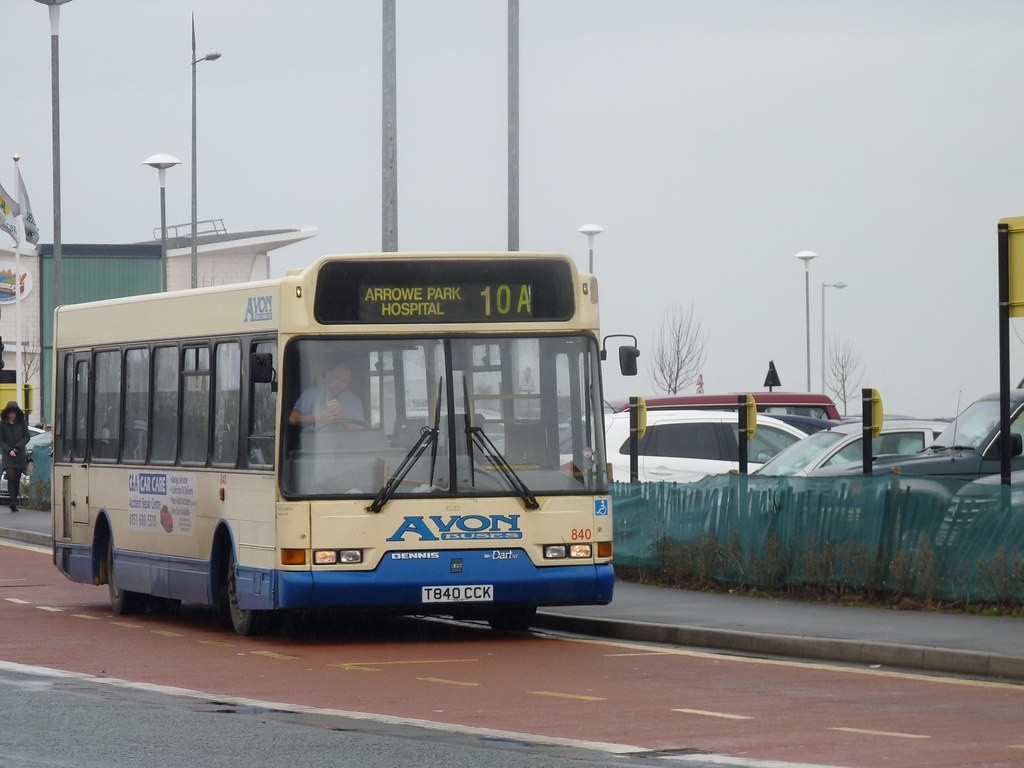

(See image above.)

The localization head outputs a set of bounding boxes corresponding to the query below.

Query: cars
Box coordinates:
[369,381,1024,552]
[0,426,53,501]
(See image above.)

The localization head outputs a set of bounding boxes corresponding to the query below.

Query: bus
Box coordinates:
[52,249,640,639]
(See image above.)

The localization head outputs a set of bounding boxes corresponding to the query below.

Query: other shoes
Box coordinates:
[10,505,19,512]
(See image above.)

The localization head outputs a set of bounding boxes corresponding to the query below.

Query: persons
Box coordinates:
[0,401,30,511]
[289,360,365,432]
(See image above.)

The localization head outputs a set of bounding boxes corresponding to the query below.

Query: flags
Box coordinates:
[0,184,27,248]
[16,166,40,246]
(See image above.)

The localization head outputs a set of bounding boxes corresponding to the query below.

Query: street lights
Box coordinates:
[819,281,852,392]
[578,224,606,273]
[796,250,821,392]
[191,10,223,290]
[142,153,182,293]
